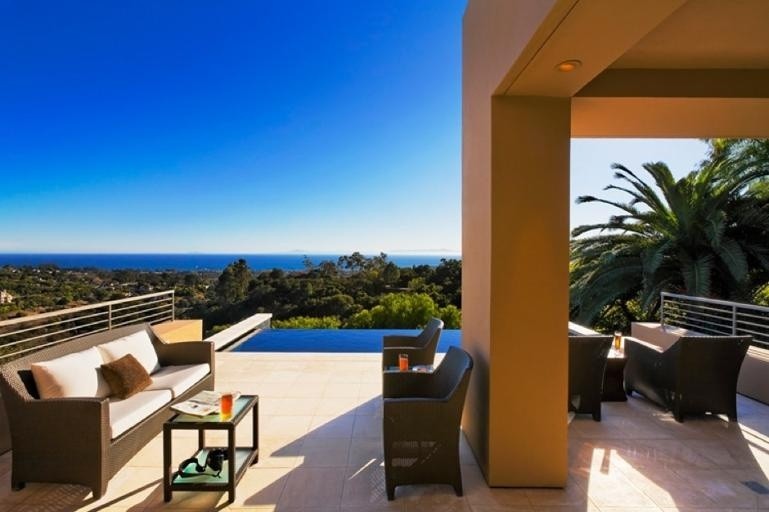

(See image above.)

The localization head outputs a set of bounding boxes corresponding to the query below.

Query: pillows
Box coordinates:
[29,328,163,400]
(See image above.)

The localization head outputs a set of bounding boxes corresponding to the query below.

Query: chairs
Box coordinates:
[568,334,753,424]
[381,316,473,501]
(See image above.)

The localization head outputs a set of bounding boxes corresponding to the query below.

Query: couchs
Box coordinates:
[0,322,216,500]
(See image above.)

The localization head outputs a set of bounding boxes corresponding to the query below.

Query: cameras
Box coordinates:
[176,447,231,478]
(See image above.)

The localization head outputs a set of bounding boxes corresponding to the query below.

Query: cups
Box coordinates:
[219,392,233,421]
[613,331,621,350]
[398,353,409,372]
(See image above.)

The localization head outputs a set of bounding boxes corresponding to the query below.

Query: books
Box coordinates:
[169,389,241,417]
